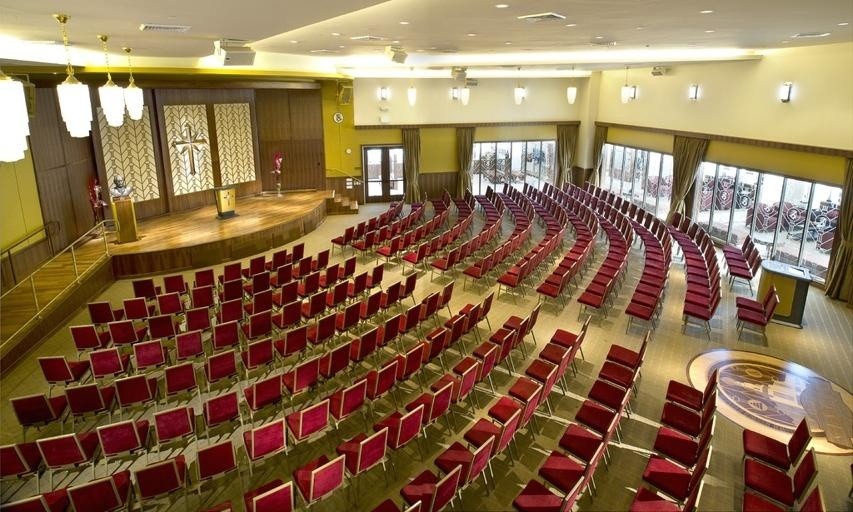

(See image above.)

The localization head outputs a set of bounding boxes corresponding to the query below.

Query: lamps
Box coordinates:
[125,48,144,123]
[0,69,30,163]
[97,34,125,128]
[56,13,94,140]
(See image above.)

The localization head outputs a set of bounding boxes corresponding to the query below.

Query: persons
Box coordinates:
[112,175,131,198]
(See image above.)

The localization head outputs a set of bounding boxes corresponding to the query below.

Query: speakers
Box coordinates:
[385,44,408,63]
[211,40,256,66]
[651,69,664,76]
